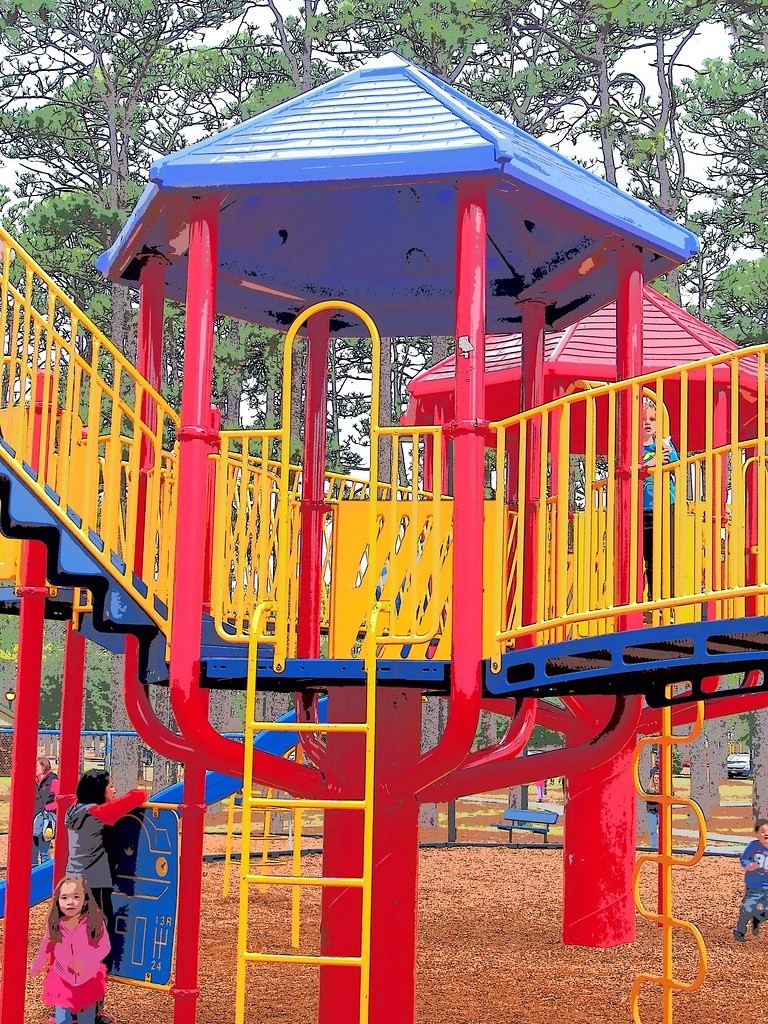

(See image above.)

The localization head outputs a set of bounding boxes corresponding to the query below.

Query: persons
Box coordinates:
[534,777,561,802]
[733,818,768,942]
[32,757,154,1024]
[642,396,680,602]
[645,755,659,848]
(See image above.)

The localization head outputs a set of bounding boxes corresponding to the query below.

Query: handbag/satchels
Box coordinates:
[33,808,57,842]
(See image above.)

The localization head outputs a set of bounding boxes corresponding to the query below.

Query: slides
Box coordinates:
[0,694,330,923]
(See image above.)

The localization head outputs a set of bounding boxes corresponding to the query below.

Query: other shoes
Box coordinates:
[536,799,542,803]
[733,929,745,942]
[752,916,760,936]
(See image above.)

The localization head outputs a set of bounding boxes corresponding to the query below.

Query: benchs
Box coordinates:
[490,808,559,843]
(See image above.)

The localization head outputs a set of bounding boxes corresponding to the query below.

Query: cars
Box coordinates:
[727,753,751,778]
[682,756,692,768]
